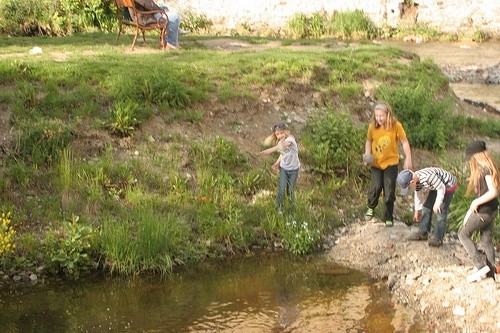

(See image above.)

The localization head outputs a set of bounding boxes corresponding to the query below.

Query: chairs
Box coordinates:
[113,0,168,52]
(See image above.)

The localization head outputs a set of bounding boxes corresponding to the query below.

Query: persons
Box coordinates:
[255,123,300,216]
[363,101,412,226]
[128,0,182,50]
[122,6,190,35]
[458,140,500,282]
[398,167,457,247]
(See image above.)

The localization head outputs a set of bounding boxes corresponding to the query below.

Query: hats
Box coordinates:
[272,122,289,132]
[463,140,486,162]
[397,170,412,195]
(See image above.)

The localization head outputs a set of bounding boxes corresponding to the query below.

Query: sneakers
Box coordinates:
[466,266,490,283]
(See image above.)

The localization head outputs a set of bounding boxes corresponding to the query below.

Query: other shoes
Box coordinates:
[160,40,167,47]
[177,27,190,35]
[428,238,443,246]
[408,231,428,240]
[166,42,182,49]
[365,205,374,216]
[386,220,393,226]
